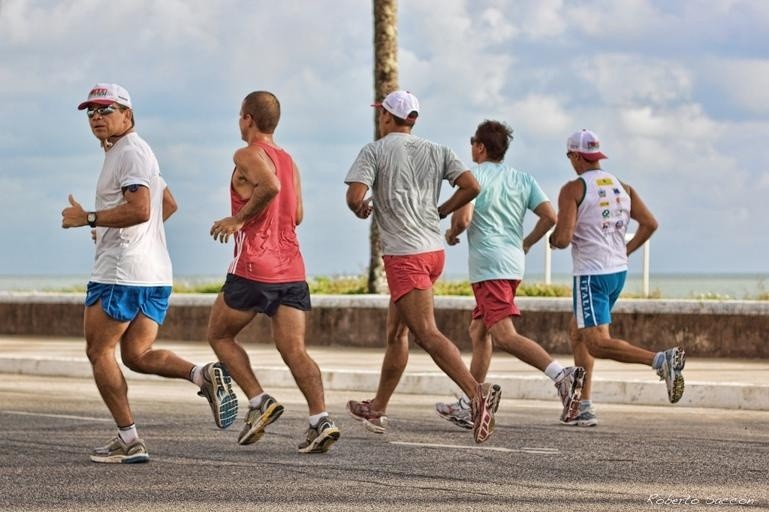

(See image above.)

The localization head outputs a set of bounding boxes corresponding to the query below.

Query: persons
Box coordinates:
[60,83,239,464]
[549,128,686,427]
[346,88,501,444]
[207,91,342,455]
[434,119,586,431]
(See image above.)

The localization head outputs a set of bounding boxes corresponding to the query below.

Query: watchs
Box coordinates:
[86,211,98,228]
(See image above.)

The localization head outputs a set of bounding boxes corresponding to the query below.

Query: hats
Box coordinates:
[78,81,133,111]
[369,90,420,121]
[566,128,608,163]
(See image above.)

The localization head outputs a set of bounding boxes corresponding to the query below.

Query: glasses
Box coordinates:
[471,135,483,147]
[85,106,124,117]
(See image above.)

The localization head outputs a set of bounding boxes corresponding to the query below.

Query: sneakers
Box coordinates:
[656,345,687,406]
[434,398,475,430]
[559,399,597,427]
[194,360,241,431]
[89,434,149,466]
[237,393,285,448]
[346,398,389,435]
[296,416,341,455]
[553,365,588,424]
[468,382,503,444]
